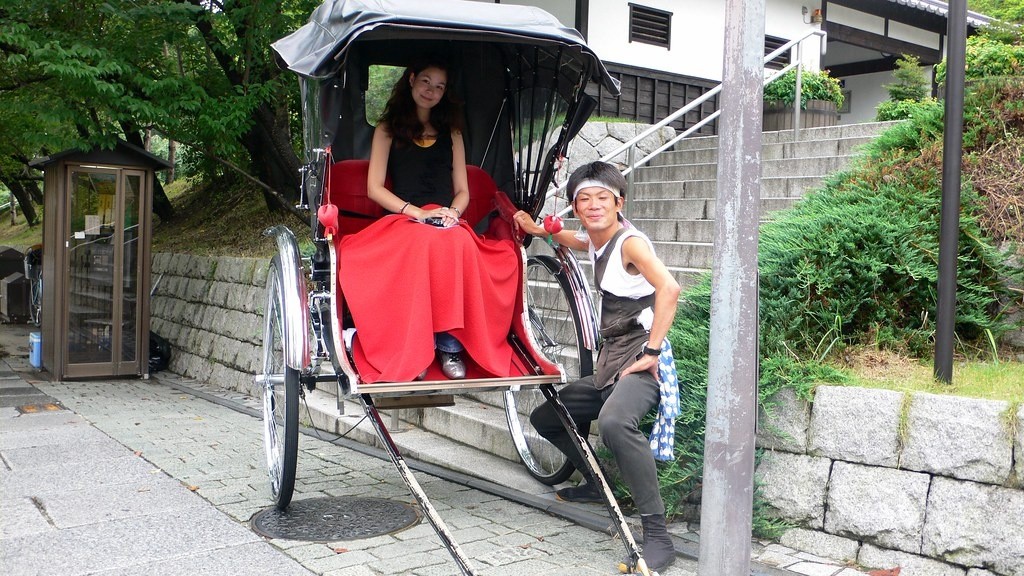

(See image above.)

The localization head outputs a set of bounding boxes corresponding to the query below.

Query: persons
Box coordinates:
[341,53,519,383]
[513,160,682,572]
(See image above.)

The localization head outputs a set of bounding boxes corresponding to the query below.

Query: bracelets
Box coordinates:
[400,202,410,214]
[450,206,461,217]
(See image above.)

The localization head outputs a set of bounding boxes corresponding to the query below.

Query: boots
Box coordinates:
[640,512,677,573]
[555,464,617,504]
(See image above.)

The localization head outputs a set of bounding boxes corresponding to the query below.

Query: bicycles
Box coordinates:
[23,245,42,327]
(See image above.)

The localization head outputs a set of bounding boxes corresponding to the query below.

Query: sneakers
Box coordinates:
[440,351,466,379]
[416,368,426,380]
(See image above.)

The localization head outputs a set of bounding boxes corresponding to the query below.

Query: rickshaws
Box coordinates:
[253,0,651,576]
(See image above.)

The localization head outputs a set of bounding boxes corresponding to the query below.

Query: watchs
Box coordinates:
[641,340,662,356]
